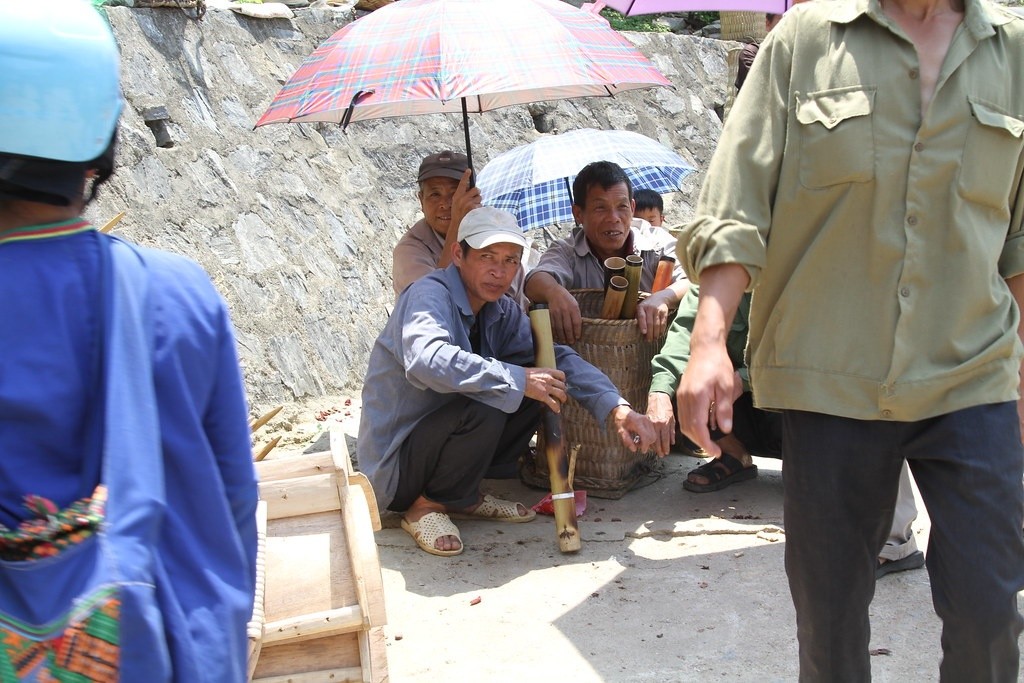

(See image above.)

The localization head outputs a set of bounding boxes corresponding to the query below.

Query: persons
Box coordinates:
[632,189,669,232]
[735,13,782,91]
[645,284,782,492]
[356,206,656,555]
[875,457,925,580]
[676,0,1024,682]
[0,0,260,683]
[523,161,692,344]
[392,149,530,316]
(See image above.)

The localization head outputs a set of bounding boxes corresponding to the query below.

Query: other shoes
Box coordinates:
[482,449,539,480]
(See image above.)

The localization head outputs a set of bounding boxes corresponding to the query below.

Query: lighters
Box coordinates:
[633,435,641,445]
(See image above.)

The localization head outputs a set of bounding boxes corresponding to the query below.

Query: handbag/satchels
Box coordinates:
[0,229,179,683]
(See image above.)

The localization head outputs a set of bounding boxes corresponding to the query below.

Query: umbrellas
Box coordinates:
[600,0,794,19]
[252,0,676,200]
[475,127,699,231]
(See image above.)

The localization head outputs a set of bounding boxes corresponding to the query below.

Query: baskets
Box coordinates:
[719,10,767,43]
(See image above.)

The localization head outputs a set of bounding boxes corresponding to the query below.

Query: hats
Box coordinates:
[416,148,469,180]
[456,206,530,250]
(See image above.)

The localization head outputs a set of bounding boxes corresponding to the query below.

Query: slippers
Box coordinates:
[682,452,759,493]
[400,511,464,557]
[873,550,925,581]
[452,494,537,523]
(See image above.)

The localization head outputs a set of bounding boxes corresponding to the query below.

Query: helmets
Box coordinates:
[0,0,130,164]
